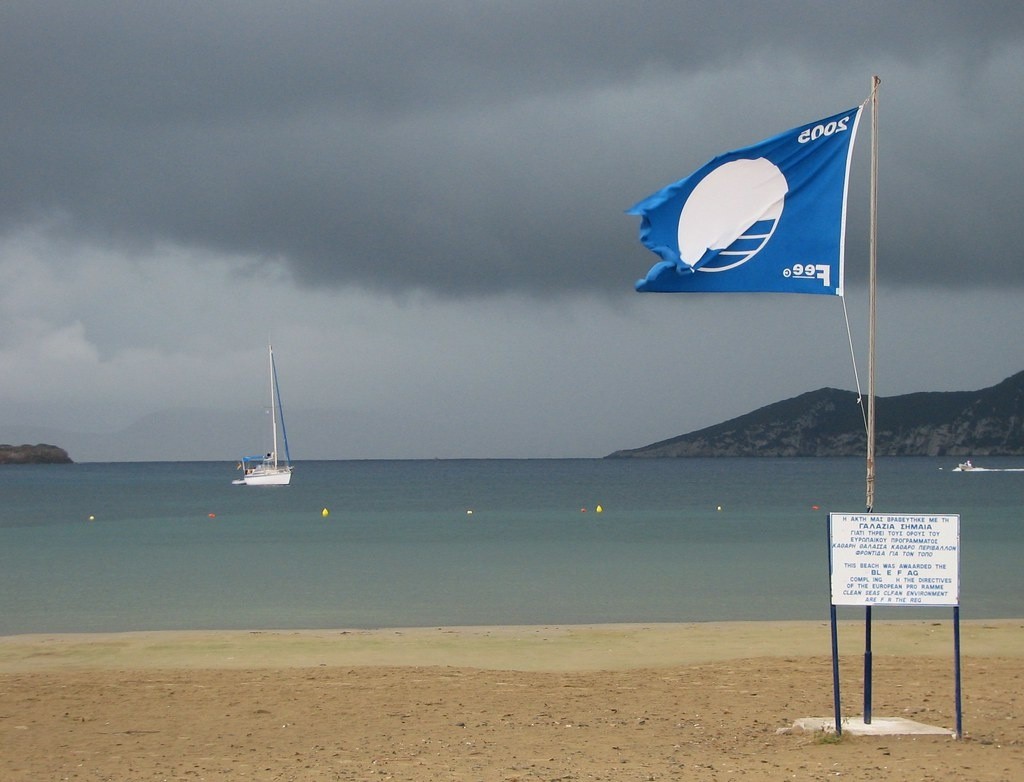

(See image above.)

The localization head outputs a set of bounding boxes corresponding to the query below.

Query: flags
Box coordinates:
[622,103,864,296]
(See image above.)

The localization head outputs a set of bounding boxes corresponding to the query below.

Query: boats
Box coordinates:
[956,460,976,473]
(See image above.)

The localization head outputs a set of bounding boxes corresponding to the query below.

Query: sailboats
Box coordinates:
[234,343,298,485]
